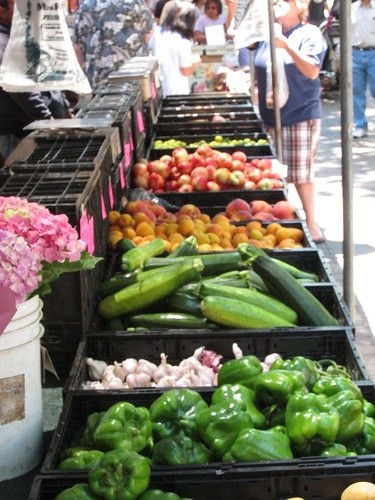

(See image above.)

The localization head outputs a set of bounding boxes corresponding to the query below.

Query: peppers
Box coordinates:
[52,355,375,500]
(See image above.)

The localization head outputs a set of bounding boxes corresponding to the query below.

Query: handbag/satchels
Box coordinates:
[265,23,289,110]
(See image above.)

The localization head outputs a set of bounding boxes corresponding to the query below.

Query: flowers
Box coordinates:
[0,196,105,336]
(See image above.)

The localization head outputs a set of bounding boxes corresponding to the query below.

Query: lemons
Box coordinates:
[152,135,269,149]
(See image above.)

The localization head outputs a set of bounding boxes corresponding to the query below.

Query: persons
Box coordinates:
[332,0,375,138]
[64,0,154,88]
[0,0,83,167]
[246,0,327,243]
[147,0,344,100]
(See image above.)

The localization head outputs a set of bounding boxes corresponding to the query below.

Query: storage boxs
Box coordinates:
[0,59,375,500]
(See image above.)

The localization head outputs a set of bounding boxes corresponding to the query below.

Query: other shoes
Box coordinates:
[313,236,327,244]
[352,128,368,138]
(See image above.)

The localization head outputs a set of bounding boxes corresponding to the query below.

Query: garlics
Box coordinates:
[78,342,282,389]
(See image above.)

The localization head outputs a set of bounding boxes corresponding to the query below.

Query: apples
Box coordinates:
[131,144,284,192]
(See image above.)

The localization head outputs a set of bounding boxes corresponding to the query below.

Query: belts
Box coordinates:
[352,45,375,52]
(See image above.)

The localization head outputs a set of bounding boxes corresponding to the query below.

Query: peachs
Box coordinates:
[107,197,306,251]
